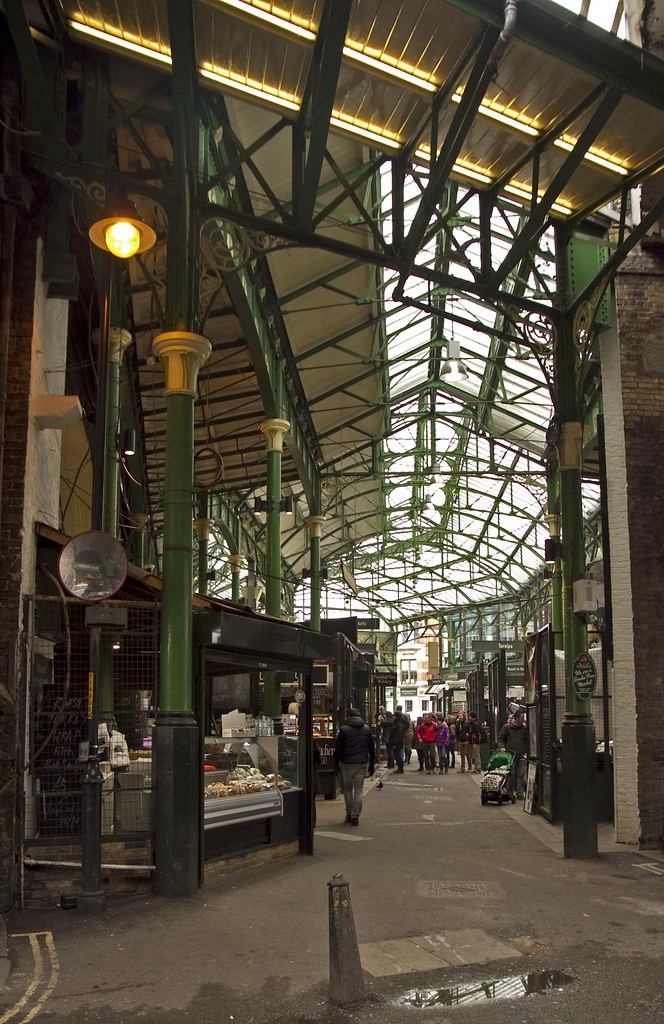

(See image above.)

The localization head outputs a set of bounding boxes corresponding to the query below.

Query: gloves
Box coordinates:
[335,766,340,777]
[367,765,374,776]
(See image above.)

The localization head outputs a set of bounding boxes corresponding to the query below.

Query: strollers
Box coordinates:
[479,749,518,806]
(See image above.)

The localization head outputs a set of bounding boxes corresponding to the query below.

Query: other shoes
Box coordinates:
[426,769,431,774]
[405,759,407,763]
[439,769,443,774]
[471,768,482,774]
[384,764,394,768]
[407,760,410,764]
[449,765,454,768]
[457,769,465,773]
[432,769,436,774]
[467,768,473,773]
[417,766,424,771]
[350,818,359,826]
[517,794,525,800]
[346,814,351,823]
[445,769,448,773]
[394,767,404,773]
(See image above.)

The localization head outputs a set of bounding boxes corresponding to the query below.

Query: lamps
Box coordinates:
[543,569,552,581]
[254,498,268,515]
[302,568,310,579]
[89,200,157,260]
[280,496,293,516]
[319,569,328,582]
[208,571,216,583]
[117,429,136,456]
[239,597,245,605]
[545,539,561,564]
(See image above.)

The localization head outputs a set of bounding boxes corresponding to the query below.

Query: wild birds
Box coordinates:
[375,781,383,791]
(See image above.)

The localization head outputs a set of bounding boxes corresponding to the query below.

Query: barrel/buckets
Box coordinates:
[479,742,489,770]
[380,748,387,761]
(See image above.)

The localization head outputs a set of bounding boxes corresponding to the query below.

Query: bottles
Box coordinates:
[247,714,274,737]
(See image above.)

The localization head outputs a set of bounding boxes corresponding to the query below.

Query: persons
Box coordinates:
[377,705,485,773]
[334,708,375,827]
[303,740,321,828]
[497,711,530,800]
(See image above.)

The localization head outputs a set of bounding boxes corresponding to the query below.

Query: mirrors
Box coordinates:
[58,532,128,602]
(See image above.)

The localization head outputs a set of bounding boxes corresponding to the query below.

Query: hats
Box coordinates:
[345,708,360,716]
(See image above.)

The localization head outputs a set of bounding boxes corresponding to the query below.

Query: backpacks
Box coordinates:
[480,726,487,743]
